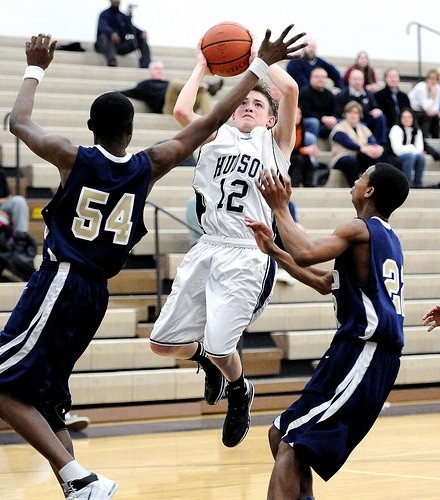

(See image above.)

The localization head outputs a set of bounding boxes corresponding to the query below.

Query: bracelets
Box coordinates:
[22,66,45,84]
[248,57,269,78]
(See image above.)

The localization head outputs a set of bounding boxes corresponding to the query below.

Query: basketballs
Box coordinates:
[203,21,252,76]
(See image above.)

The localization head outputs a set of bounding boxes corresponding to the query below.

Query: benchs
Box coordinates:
[0,37,440,408]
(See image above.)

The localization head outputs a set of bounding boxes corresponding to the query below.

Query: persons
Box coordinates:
[0,146,30,238]
[120,62,223,116]
[150,29,298,448]
[0,24,309,500]
[271,41,440,188]
[95,0,150,68]
[187,195,201,246]
[273,201,307,285]
[244,163,409,500]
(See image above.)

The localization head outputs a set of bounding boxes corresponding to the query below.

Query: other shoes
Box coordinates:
[2,253,37,282]
[64,412,88,432]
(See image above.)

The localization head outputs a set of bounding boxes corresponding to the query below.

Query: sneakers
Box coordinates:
[63,472,118,500]
[222,377,255,447]
[195,357,224,405]
